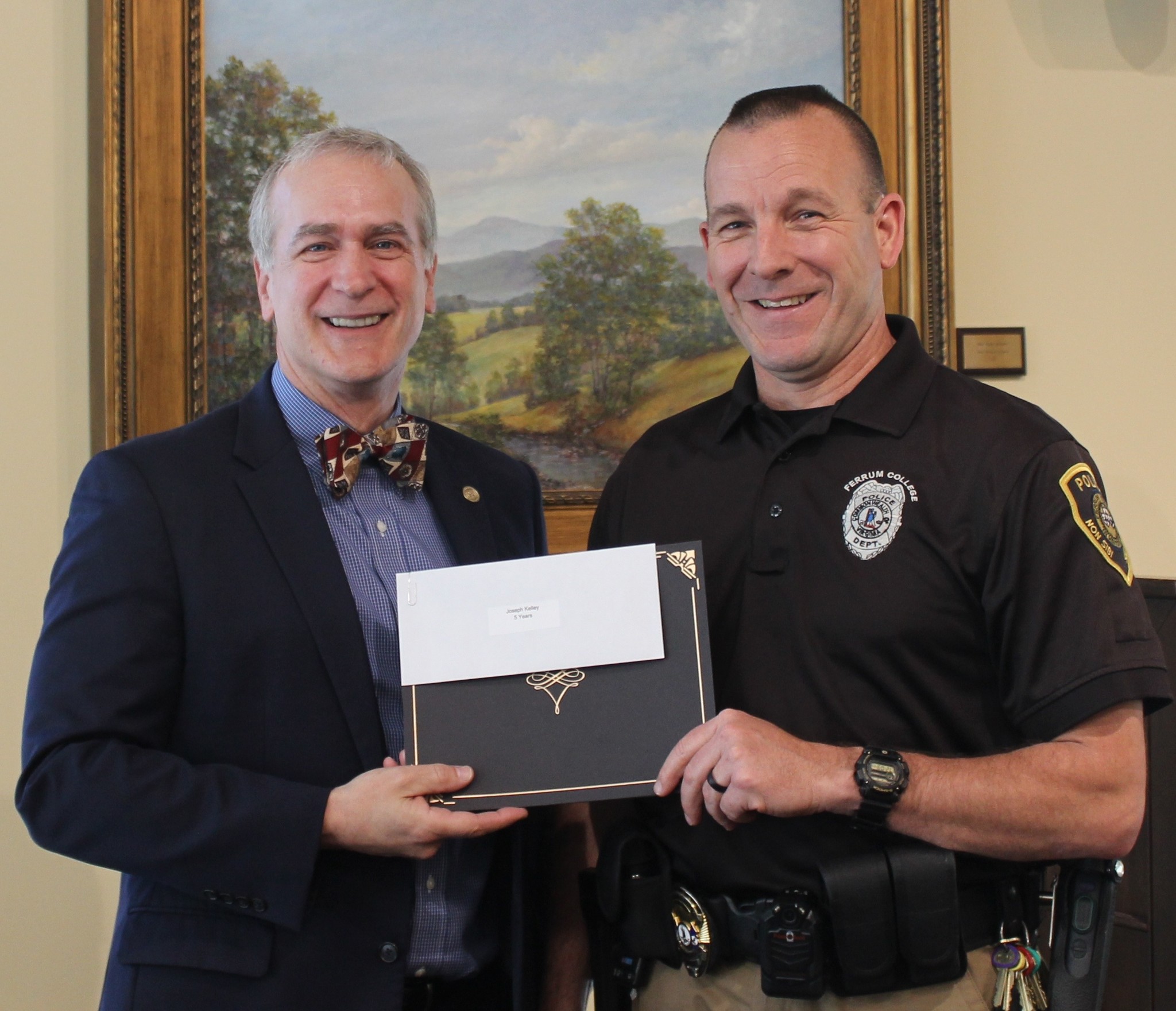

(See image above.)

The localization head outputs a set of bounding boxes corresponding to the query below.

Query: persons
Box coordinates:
[12,127,567,1011]
[583,84,1176,1011]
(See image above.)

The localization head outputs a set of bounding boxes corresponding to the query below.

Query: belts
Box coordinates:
[402,976,510,1011]
[655,876,1047,981]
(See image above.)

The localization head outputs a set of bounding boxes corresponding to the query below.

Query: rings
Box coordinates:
[707,767,728,794]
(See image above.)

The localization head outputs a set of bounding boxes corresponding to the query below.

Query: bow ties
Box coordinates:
[314,414,428,502]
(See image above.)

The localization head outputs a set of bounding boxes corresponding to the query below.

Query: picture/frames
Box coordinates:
[102,0,958,556]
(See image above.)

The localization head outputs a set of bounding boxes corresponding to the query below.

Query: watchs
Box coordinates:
[853,744,910,824]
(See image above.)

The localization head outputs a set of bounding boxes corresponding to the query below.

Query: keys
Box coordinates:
[991,938,1048,1011]
